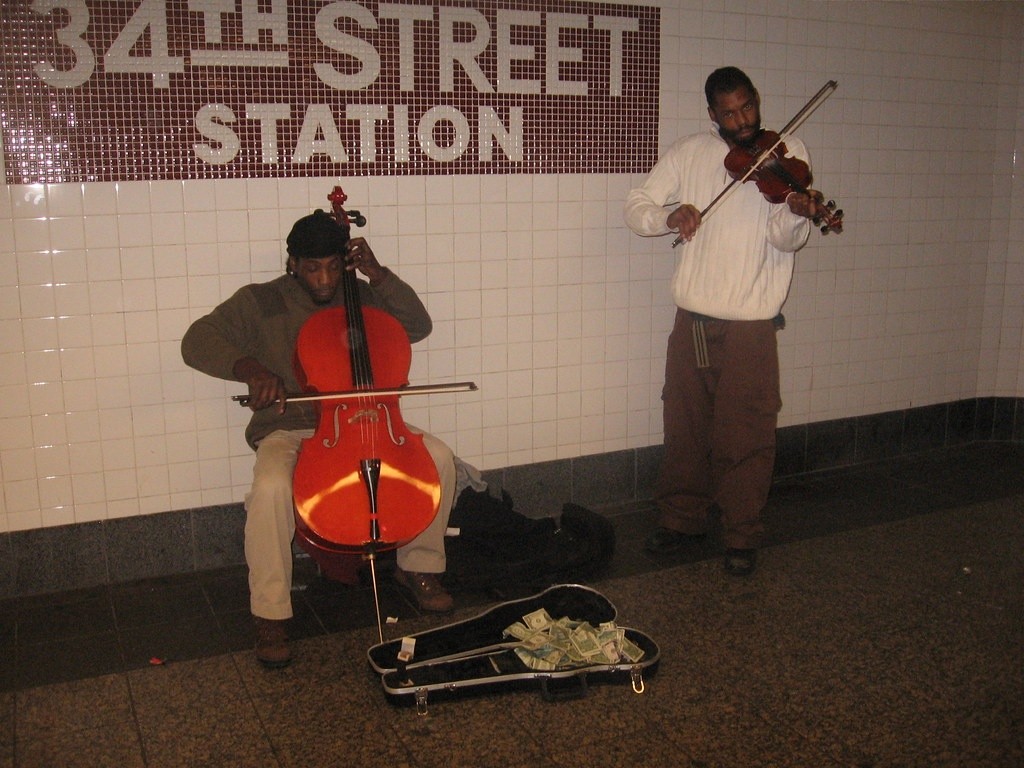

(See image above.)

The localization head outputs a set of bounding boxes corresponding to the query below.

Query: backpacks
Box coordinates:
[456,484,582,603]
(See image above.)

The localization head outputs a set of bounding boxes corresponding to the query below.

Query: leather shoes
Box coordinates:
[649,527,693,554]
[252,617,290,670]
[724,546,758,569]
[396,565,456,613]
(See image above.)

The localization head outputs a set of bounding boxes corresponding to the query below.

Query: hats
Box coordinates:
[286,209,352,258]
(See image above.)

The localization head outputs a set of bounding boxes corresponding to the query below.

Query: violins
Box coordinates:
[723,128,844,235]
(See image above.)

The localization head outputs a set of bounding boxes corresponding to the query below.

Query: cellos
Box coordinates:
[287,185,443,643]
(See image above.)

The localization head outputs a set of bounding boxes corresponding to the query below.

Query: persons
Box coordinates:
[181,209,457,664]
[625,66,824,574]
